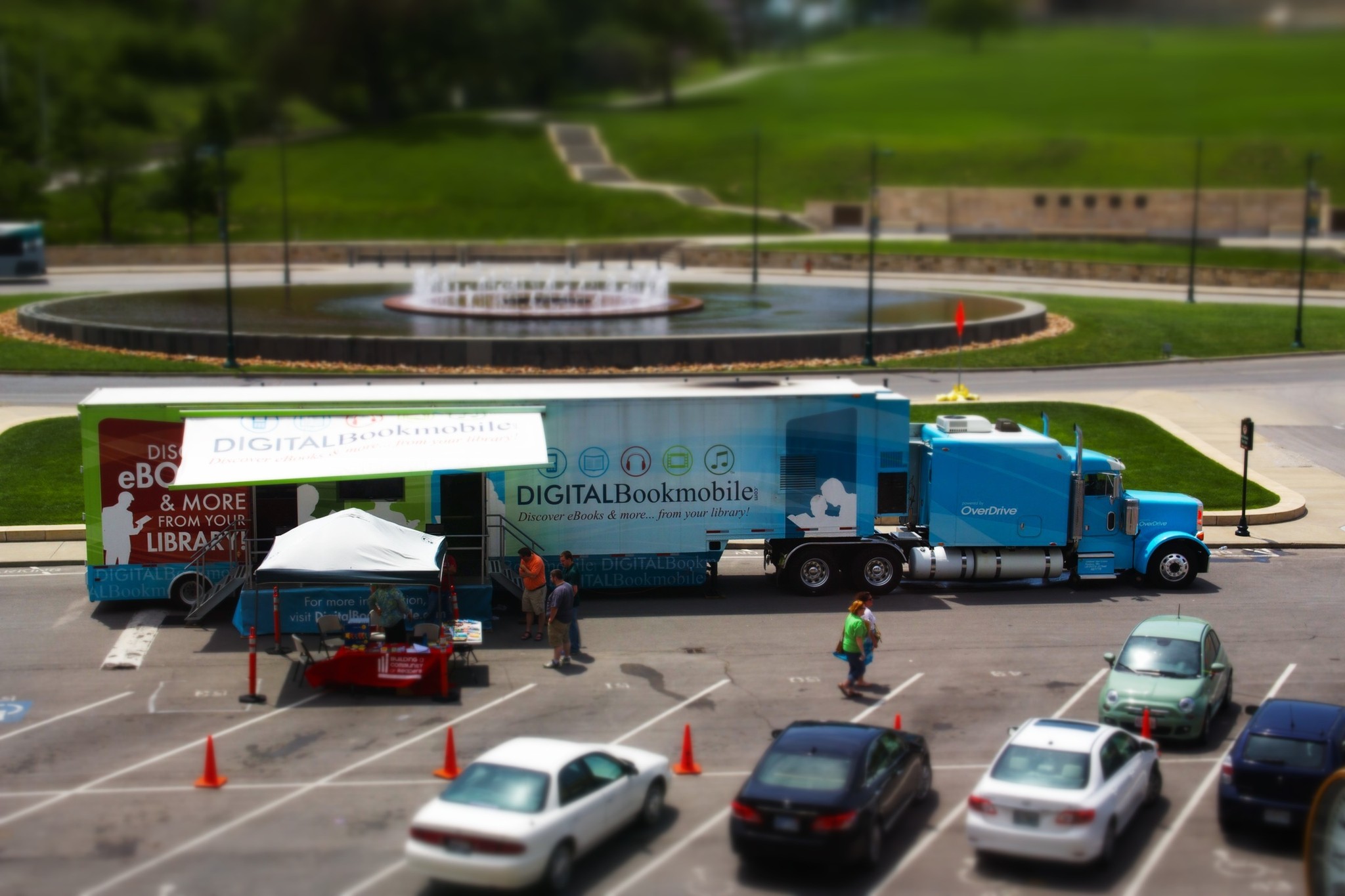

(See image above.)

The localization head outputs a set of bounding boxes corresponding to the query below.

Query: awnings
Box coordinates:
[165,412,554,490]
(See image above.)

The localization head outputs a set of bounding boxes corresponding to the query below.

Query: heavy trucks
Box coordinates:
[80,375,1212,614]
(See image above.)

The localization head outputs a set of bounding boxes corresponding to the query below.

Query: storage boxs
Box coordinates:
[345,617,370,645]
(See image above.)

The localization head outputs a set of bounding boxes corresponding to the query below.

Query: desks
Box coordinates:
[305,642,452,700]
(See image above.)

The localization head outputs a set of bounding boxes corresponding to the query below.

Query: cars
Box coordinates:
[401,736,674,896]
[1098,612,1233,747]
[725,719,934,886]
[964,717,1164,875]
[1219,697,1345,847]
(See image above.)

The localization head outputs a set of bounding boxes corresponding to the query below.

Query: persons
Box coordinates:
[518,547,547,641]
[426,555,457,624]
[542,569,574,668]
[367,586,414,642]
[837,591,878,696]
[560,550,580,654]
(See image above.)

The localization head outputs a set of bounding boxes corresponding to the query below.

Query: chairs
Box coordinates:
[317,614,344,658]
[412,623,439,644]
[292,634,316,687]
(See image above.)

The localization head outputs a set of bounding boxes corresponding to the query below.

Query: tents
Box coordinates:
[254,507,449,635]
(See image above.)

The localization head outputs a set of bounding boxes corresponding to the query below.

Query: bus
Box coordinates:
[0,220,47,277]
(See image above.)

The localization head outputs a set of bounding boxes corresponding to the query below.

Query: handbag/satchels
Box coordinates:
[836,640,844,653]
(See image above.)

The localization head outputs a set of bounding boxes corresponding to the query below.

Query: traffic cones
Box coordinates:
[1140,707,1155,740]
[672,722,703,775]
[893,712,903,730]
[431,727,466,779]
[192,734,227,787]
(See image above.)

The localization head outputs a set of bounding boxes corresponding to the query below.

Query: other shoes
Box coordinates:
[839,683,852,698]
[857,682,871,687]
[570,649,580,655]
[544,659,560,668]
[561,658,569,663]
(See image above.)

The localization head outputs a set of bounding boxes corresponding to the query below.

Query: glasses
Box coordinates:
[867,597,873,601]
[859,607,866,610]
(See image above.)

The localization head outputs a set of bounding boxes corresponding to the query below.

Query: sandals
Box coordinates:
[535,632,542,641]
[521,632,531,639]
